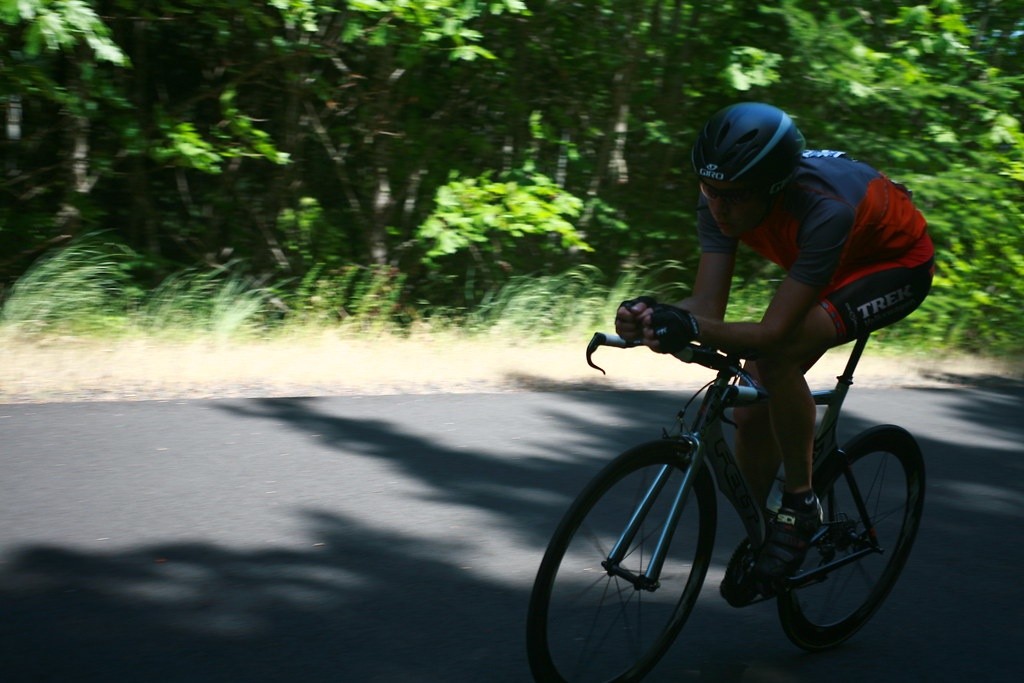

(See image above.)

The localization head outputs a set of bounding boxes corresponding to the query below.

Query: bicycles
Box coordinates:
[525,330,926,683]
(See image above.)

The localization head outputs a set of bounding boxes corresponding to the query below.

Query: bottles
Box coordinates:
[766,460,787,513]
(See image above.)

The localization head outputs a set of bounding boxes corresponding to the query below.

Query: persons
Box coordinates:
[616,104,934,603]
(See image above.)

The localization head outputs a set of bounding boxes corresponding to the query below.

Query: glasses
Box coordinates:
[696,176,760,206]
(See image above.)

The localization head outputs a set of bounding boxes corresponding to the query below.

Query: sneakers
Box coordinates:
[757,493,824,578]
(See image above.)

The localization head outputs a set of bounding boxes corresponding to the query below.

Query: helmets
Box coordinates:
[691,101,807,211]
